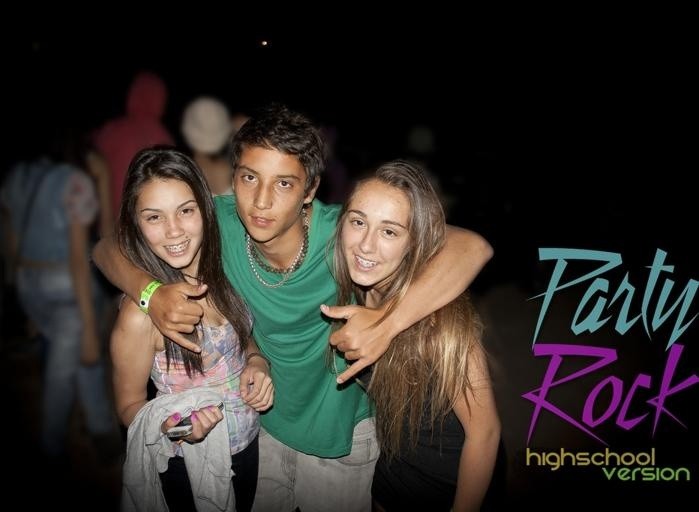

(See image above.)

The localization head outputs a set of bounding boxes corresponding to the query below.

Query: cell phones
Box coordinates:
[166,401,224,438]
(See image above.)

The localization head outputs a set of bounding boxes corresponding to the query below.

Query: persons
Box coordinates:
[0,76,233,443]
[325,158,502,512]
[110,144,276,512]
[90,104,494,512]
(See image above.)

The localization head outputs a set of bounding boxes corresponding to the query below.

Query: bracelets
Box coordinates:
[244,352,271,368]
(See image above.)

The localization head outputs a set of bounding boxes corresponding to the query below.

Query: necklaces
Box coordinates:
[245,207,310,289]
